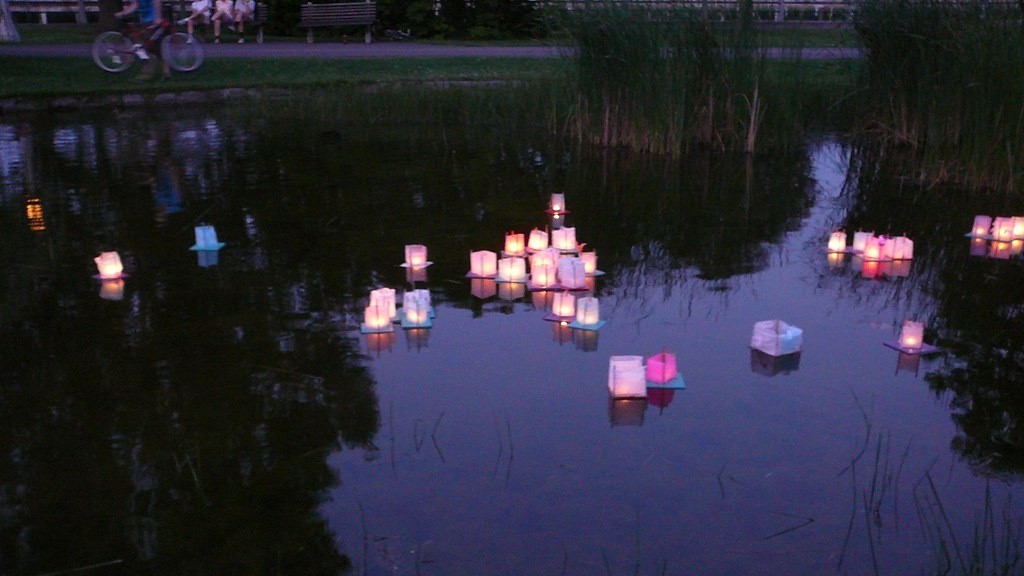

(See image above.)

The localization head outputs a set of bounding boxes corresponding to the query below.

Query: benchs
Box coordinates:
[194,2,266,45]
[300,2,377,46]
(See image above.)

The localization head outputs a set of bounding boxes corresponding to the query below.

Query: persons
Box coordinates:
[177,0,256,44]
[113,0,171,81]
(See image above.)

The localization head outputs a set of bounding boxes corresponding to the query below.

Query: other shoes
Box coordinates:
[186,35,193,43]
[234,20,240,26]
[238,37,244,43]
[177,19,187,25]
[214,38,220,43]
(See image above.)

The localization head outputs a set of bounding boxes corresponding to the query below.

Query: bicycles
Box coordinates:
[92,14,204,74]
